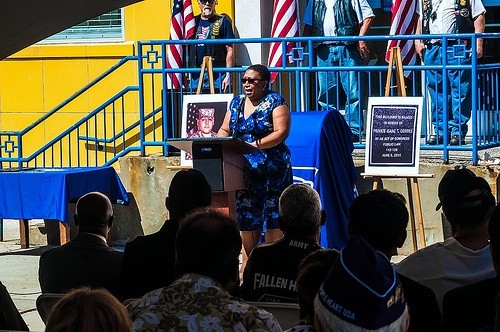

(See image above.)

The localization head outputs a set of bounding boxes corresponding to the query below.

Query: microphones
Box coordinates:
[234,96,245,138]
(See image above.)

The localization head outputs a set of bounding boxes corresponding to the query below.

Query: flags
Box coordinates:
[384,0,420,82]
[166,0,195,92]
[267,0,298,83]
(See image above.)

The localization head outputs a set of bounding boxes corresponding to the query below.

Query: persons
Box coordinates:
[299,0,375,142]
[0,281,31,332]
[413,0,487,146]
[181,0,233,94]
[43,289,135,332]
[214,64,291,279]
[120,169,213,305]
[238,184,341,302]
[122,207,282,332]
[185,106,220,160]
[284,165,500,332]
[36,193,125,295]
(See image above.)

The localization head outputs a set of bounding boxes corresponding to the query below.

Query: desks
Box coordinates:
[0,167,131,246]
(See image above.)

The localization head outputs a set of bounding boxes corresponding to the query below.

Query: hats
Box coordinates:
[443,177,492,215]
[436,169,476,210]
[318,241,406,330]
[199,108,215,119]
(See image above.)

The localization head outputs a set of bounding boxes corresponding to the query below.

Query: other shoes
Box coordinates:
[450,136,465,145]
[352,134,361,142]
[427,136,443,144]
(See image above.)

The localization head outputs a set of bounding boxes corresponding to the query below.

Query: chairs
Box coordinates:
[240,301,301,330]
[36,293,69,327]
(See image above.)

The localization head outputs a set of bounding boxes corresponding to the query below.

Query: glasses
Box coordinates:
[241,77,260,84]
[200,0,215,5]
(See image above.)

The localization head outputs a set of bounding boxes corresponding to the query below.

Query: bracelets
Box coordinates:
[255,138,264,150]
